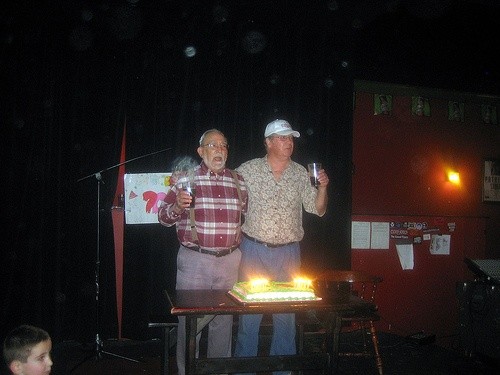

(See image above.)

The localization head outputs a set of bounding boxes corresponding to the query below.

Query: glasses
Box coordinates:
[265,135,296,141]
[200,143,230,150]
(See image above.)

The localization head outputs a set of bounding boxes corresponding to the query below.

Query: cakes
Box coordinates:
[231,280,318,302]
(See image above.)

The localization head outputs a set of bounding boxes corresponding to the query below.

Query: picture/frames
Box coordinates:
[480,158,500,205]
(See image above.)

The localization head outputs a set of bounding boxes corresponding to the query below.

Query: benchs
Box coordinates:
[148,321,319,375]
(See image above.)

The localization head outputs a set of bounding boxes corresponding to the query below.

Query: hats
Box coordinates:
[264,119,300,138]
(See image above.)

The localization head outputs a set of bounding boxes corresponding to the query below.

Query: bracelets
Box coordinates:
[171,202,186,217]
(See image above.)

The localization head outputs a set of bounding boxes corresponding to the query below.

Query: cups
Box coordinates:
[182,181,196,208]
[307,162,322,187]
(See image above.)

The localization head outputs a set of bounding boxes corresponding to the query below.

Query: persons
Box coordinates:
[157,127,249,375]
[169,119,330,375]
[0,324,53,375]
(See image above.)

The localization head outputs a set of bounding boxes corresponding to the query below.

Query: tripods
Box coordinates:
[64,145,178,375]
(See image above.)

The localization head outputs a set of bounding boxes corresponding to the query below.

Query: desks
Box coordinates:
[165,289,352,375]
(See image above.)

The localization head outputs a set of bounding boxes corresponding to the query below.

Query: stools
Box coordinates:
[318,271,384,375]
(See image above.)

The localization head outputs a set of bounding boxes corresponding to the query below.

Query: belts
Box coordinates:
[242,232,296,248]
[181,243,240,257]
